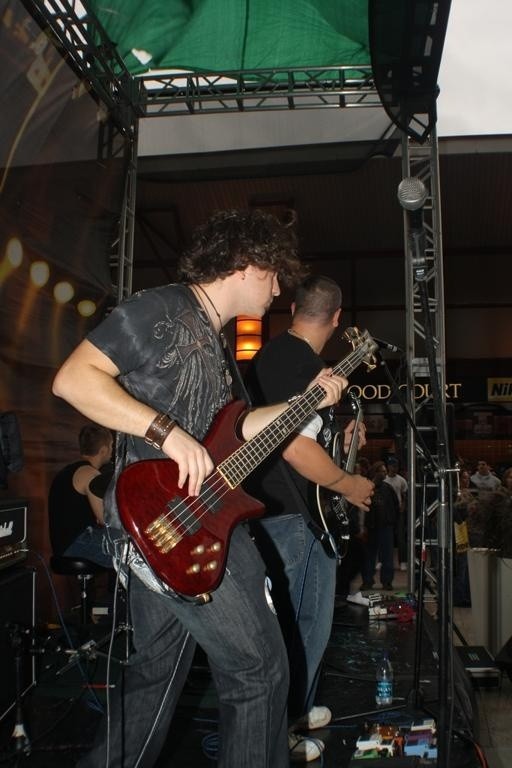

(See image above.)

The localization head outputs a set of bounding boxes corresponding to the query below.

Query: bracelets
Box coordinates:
[144,411,169,446]
[152,418,178,451]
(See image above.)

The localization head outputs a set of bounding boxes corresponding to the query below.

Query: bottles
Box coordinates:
[376,650,394,705]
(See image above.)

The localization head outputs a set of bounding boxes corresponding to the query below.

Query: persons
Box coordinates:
[52,196,352,767]
[332,444,512,593]
[236,274,375,762]
[43,418,130,570]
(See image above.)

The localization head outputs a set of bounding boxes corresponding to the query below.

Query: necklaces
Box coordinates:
[289,328,321,355]
[190,283,225,337]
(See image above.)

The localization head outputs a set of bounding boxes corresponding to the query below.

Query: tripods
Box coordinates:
[330,461,466,740]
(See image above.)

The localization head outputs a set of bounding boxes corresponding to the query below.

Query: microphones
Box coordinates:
[343,326,398,354]
[397,176,428,266]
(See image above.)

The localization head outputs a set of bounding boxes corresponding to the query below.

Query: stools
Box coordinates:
[50,556,106,647]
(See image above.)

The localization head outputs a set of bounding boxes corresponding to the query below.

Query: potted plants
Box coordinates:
[467,503,498,649]
[485,500,512,655]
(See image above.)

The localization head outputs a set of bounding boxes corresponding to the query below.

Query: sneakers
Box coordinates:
[359,582,374,591]
[381,581,393,591]
[285,730,325,761]
[287,706,331,730]
[375,562,383,570]
[347,592,370,606]
[400,561,408,571]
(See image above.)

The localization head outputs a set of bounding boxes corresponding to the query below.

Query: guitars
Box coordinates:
[308,391,365,558]
[115,327,379,604]
